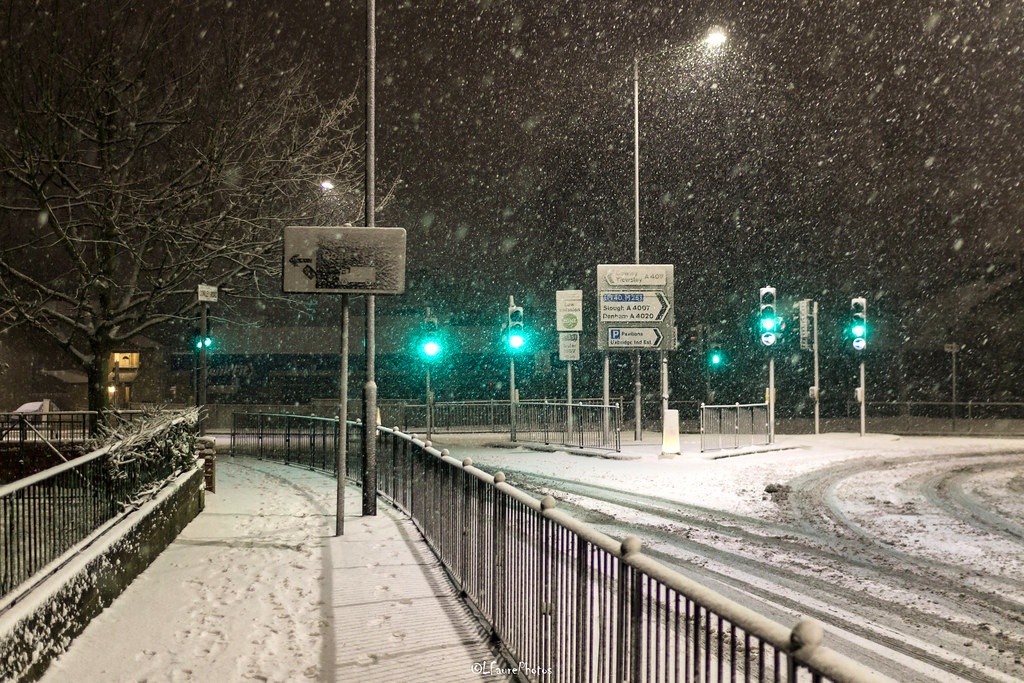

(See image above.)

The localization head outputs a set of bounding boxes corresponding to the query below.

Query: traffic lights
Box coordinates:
[508,305,526,352]
[793,298,814,351]
[850,297,867,352]
[759,286,778,346]
[710,343,722,366]
[420,318,441,362]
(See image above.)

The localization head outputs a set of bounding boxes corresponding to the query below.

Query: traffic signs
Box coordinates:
[600,289,673,323]
[606,325,664,349]
[606,267,667,286]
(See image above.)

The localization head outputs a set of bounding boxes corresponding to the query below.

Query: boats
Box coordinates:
[689,324,704,357]
